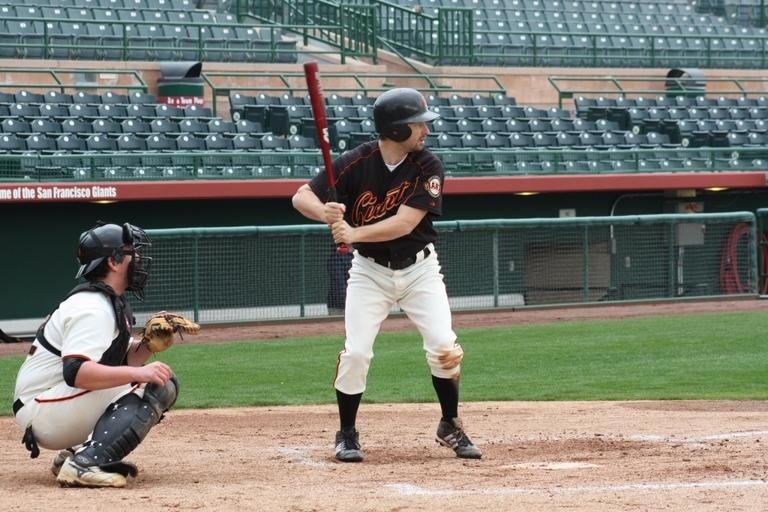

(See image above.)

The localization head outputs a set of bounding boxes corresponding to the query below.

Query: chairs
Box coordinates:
[379,1,768,67]
[0,94,767,183]
[6,2,300,60]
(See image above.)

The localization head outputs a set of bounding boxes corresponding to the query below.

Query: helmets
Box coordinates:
[74,223,149,279]
[374,87,440,141]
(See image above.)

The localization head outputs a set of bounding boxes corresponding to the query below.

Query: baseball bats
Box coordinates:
[303,60,350,255]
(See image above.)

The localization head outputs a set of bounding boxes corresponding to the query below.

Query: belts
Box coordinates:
[358,247,431,270]
[12,399,24,417]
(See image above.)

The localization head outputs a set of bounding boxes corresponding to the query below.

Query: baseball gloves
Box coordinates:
[141,310,201,353]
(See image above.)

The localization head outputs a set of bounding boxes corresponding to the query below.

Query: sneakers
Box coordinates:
[435,418,482,458]
[50,448,127,487]
[335,430,364,462]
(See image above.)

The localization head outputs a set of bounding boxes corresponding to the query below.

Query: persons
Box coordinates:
[11,220,200,489]
[289,86,483,460]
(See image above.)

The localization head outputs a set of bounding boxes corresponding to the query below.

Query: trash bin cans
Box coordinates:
[157,61,205,109]
[664,68,706,98]
